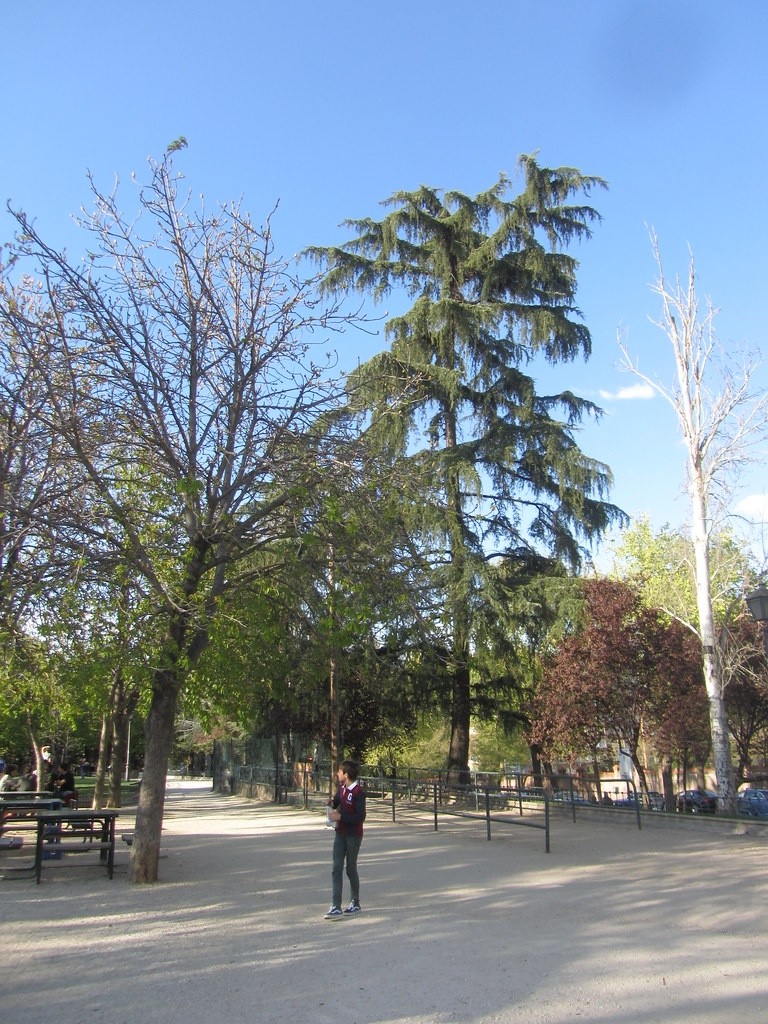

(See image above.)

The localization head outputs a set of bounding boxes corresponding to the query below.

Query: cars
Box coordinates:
[634,790,666,811]
[735,787,768,819]
[553,790,584,804]
[519,786,552,799]
[673,789,719,815]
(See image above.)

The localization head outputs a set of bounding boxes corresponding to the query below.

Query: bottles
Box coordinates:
[326,805,332,827]
[328,805,339,828]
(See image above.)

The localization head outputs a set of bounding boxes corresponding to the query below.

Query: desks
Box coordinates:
[0,798,66,860]
[0,790,54,822]
[34,809,120,885]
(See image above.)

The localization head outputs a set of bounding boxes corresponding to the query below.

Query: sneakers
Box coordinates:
[343,902,361,916]
[324,906,344,919]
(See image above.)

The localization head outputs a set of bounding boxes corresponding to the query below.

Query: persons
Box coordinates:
[0,765,21,816]
[17,767,37,816]
[54,763,75,800]
[324,760,366,919]
